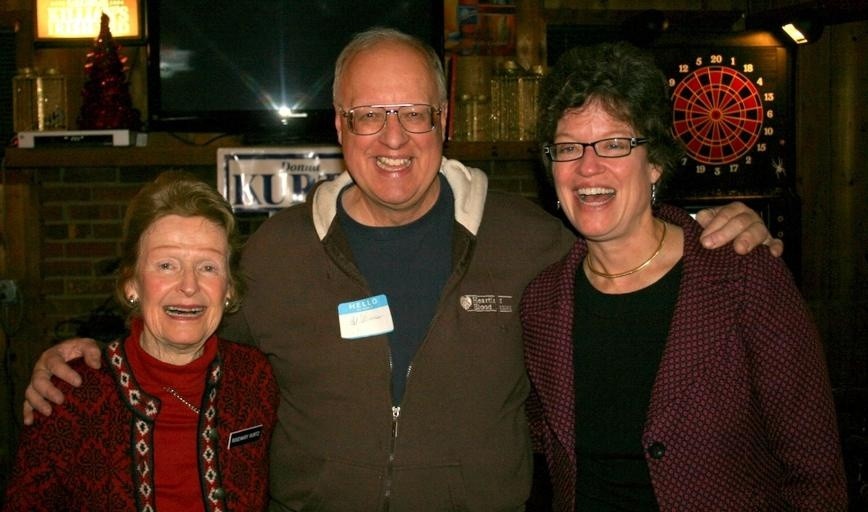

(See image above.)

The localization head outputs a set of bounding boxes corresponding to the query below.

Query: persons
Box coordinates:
[512,43,847,509]
[22,25,786,511]
[7,165,279,512]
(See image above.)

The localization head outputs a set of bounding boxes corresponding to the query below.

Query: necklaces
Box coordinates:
[587,217,669,278]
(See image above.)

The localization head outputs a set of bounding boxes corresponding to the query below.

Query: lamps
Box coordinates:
[783,19,814,43]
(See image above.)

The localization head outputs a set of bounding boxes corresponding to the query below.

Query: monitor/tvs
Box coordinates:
[30,0,150,49]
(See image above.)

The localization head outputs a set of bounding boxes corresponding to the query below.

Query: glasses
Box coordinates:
[544,137,648,162]
[342,104,440,135]
